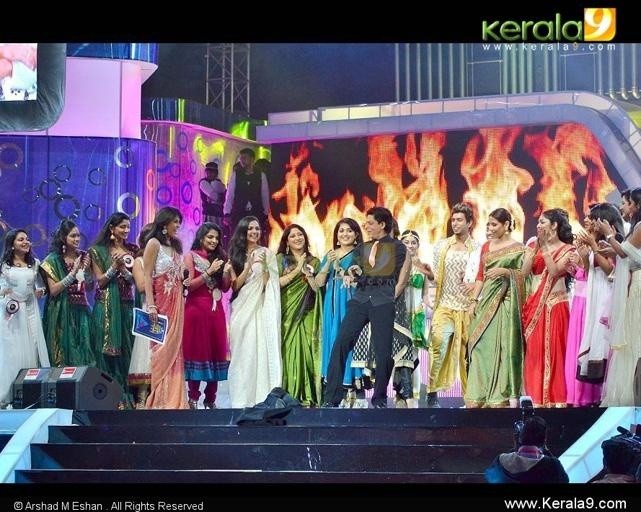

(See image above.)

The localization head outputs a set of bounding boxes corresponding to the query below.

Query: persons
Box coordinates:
[254,158,270,175]
[226,216,283,408]
[223,148,270,223]
[465,207,531,408]
[600,188,641,408]
[486,222,496,242]
[394,229,434,408]
[427,202,483,408]
[577,203,623,383]
[127,224,153,410]
[0,227,50,410]
[489,415,569,483]
[567,202,598,406]
[365,218,414,407]
[524,207,575,407]
[143,207,189,410]
[319,206,413,408]
[197,163,227,223]
[88,212,138,410]
[314,218,370,408]
[276,224,326,409]
[184,221,232,410]
[39,218,94,369]
[620,190,636,222]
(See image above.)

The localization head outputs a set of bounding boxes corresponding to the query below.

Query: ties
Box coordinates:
[368,241,380,268]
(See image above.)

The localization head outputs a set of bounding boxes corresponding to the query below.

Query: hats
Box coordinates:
[519,416,547,448]
[204,162,219,172]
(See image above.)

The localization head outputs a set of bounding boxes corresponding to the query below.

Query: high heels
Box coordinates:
[406,398,418,408]
[203,399,218,409]
[338,398,356,409]
[353,399,369,409]
[395,399,408,408]
[188,398,198,409]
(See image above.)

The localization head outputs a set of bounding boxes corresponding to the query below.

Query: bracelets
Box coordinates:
[320,267,330,274]
[121,269,132,281]
[106,266,116,280]
[200,269,210,281]
[41,287,47,295]
[469,297,479,302]
[63,273,75,286]
[541,251,553,255]
[148,304,157,308]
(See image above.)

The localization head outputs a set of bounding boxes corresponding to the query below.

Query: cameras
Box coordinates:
[512,398,534,434]
[609,424,641,453]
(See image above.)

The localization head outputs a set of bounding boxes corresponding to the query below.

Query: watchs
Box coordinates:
[337,267,343,275]
[263,268,269,273]
[603,234,615,243]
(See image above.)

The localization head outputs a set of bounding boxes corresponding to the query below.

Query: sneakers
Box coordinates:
[425,393,441,408]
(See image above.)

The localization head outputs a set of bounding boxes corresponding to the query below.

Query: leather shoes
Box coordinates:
[321,401,334,408]
[374,403,387,409]
